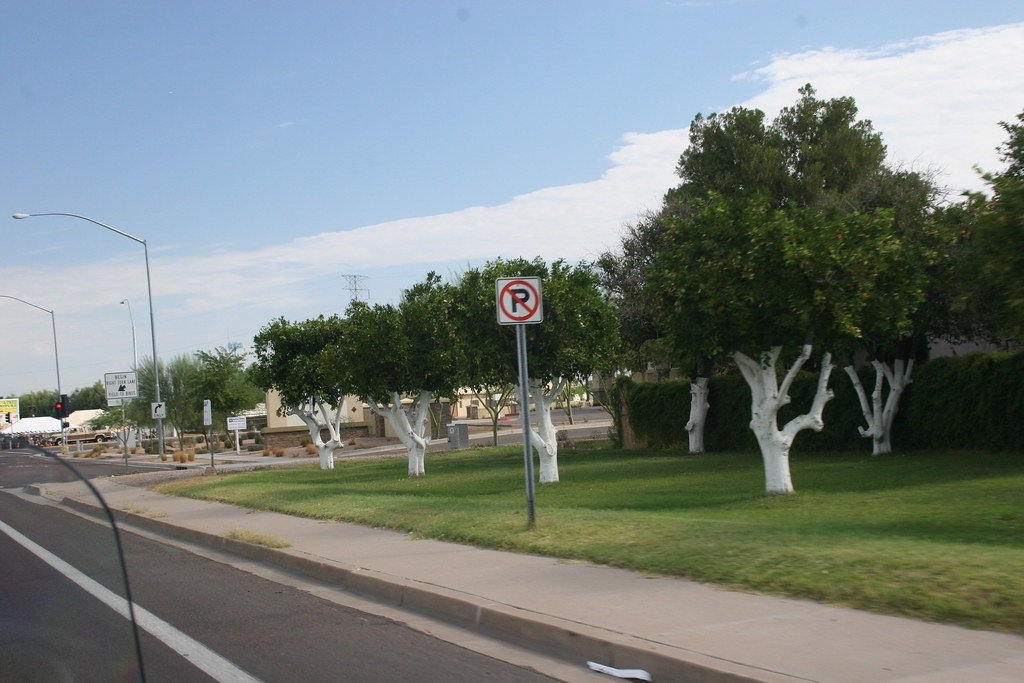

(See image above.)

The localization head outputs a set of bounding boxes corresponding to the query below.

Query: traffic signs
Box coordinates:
[105,371,140,400]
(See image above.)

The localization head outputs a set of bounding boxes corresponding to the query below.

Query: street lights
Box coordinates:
[120,298,142,447]
[12,211,164,460]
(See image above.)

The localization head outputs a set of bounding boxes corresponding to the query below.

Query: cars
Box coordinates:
[184,425,208,435]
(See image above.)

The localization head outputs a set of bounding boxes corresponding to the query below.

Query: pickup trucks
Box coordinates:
[52,427,116,446]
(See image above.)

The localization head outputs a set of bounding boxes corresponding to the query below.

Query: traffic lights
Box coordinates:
[5,412,10,423]
[55,402,62,410]
